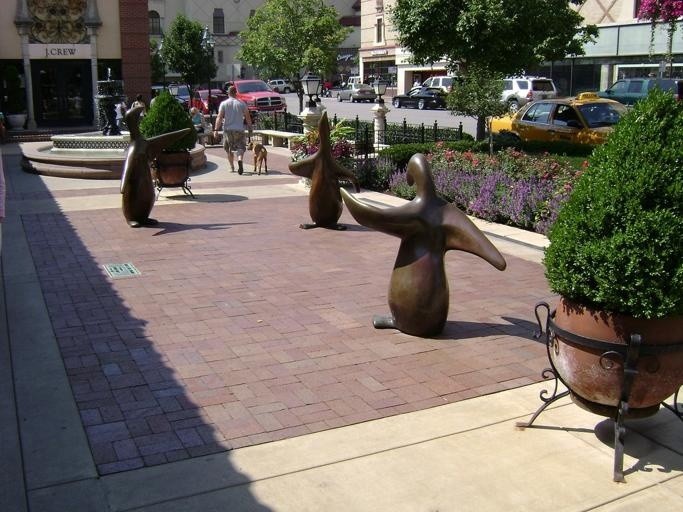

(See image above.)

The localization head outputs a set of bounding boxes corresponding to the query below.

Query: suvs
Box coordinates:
[487,73,562,116]
[340,76,361,87]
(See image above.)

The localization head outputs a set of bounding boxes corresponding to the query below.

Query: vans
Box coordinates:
[595,76,682,107]
[419,76,466,92]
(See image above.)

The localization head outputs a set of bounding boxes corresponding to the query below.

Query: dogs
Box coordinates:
[247,142,268,175]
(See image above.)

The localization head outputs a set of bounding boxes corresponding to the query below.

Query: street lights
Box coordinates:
[158,41,168,90]
[199,23,216,118]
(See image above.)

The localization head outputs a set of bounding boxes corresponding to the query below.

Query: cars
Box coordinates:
[335,83,377,104]
[151,81,227,117]
[483,91,628,150]
[265,78,294,94]
[391,86,450,109]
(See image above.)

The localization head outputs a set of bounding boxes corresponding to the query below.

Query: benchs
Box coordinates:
[197,128,304,150]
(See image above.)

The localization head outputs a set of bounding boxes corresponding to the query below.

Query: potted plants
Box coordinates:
[540,82,682,418]
[134,91,198,187]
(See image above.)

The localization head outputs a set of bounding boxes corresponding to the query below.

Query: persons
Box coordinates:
[214,85,254,176]
[412,79,421,86]
[113,90,205,145]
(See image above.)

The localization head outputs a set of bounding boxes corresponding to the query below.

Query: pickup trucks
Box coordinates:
[207,79,287,116]
